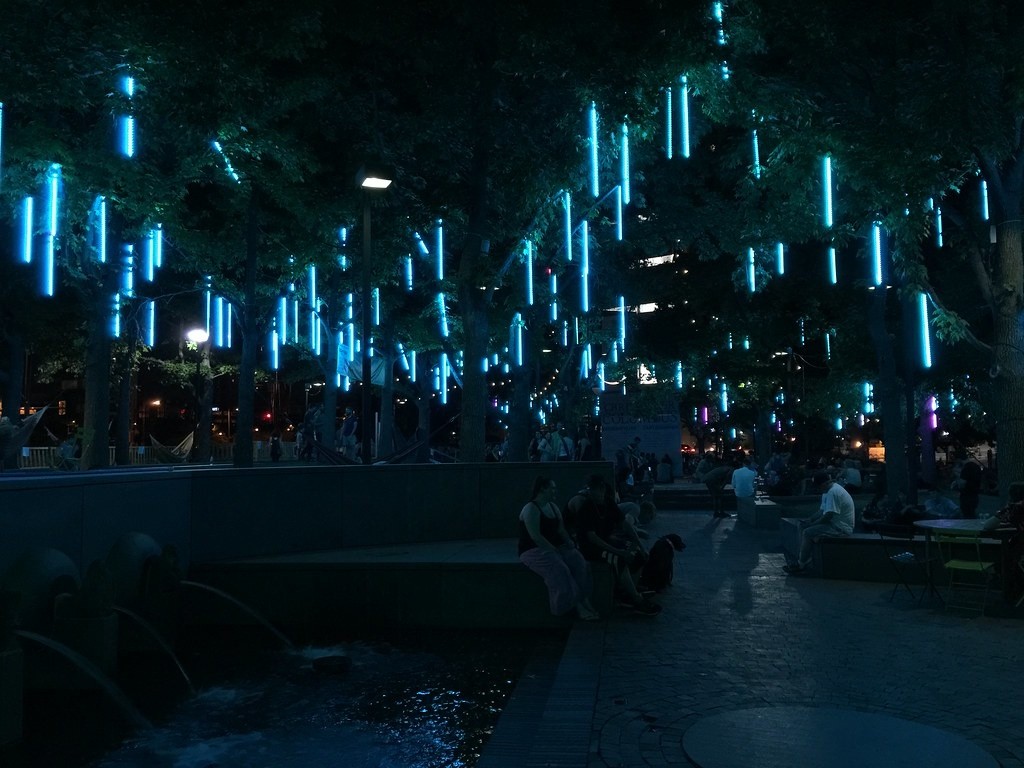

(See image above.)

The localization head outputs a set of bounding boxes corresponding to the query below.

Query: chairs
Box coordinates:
[877,522,946,607]
[930,527,1002,614]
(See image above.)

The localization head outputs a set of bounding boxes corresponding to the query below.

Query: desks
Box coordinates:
[913,519,1020,603]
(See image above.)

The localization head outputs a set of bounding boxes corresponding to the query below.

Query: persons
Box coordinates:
[302,403,325,463]
[486,422,1024,620]
[339,406,359,460]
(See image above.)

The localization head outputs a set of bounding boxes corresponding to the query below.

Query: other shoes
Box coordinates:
[714,512,731,518]
[577,606,601,621]
[633,604,658,616]
[613,596,634,608]
[788,557,813,575]
